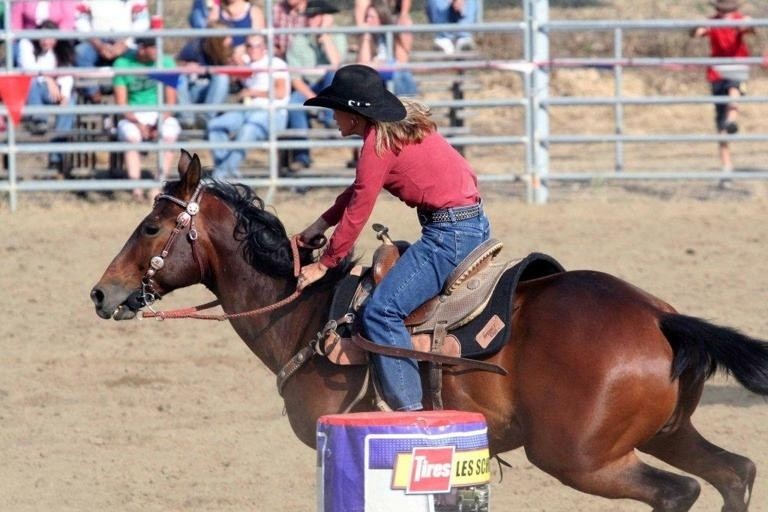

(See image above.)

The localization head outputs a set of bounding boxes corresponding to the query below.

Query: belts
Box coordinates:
[418,205,479,224]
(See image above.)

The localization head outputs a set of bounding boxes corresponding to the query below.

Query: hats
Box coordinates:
[299,0,340,17]
[710,0,745,12]
[302,64,408,123]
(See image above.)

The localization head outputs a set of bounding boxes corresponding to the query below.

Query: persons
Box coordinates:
[686,1,764,195]
[0,0,486,206]
[285,59,492,415]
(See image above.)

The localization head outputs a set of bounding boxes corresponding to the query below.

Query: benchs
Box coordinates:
[0,0,481,202]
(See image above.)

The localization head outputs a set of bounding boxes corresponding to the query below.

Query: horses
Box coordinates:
[89,148,767,512]
